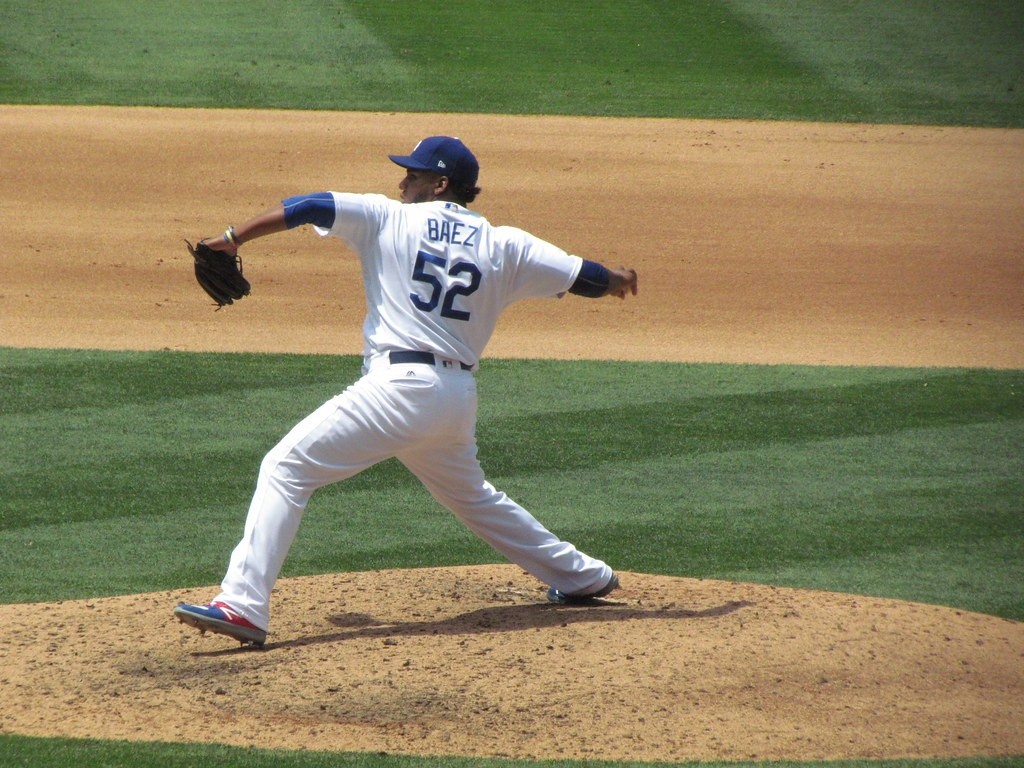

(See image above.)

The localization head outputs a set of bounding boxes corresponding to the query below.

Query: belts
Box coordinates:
[388,350,472,371]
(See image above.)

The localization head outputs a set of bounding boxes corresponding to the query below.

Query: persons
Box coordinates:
[173,136,637,646]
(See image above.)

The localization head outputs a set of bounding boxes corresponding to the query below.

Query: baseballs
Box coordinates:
[622,287,632,295]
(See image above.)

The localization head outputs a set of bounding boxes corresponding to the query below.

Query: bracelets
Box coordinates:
[224,225,243,249]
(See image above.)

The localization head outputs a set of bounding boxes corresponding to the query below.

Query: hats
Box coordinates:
[388,136,479,179]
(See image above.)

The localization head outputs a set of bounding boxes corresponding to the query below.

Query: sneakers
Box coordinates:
[546,570,618,605]
[173,601,266,646]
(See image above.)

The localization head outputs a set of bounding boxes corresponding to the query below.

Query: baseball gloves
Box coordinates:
[183,238,250,313]
